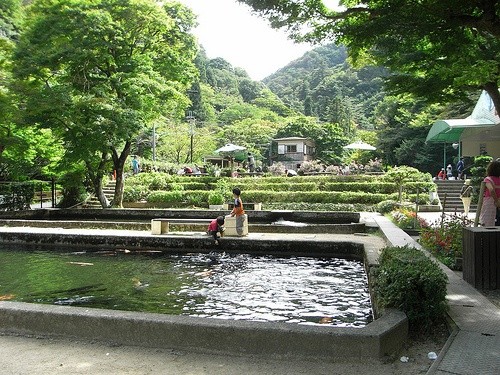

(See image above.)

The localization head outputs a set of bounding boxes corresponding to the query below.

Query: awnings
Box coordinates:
[425,117,495,144]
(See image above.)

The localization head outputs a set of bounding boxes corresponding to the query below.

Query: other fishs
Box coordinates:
[67,261,94,267]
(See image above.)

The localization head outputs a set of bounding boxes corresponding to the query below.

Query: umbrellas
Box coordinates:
[214,144,247,168]
[343,140,377,170]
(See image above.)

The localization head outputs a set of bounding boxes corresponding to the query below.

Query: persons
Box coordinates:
[285,154,387,177]
[132,155,139,176]
[232,169,241,178]
[206,215,226,246]
[436,156,500,229]
[178,163,208,177]
[244,152,255,172]
[230,187,248,237]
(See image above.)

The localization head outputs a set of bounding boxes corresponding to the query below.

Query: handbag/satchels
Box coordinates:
[460,194,463,199]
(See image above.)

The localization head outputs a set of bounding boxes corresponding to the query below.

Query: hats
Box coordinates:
[447,164,452,168]
[464,180,470,185]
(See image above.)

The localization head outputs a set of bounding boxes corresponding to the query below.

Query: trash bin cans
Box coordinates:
[463,225,500,292]
